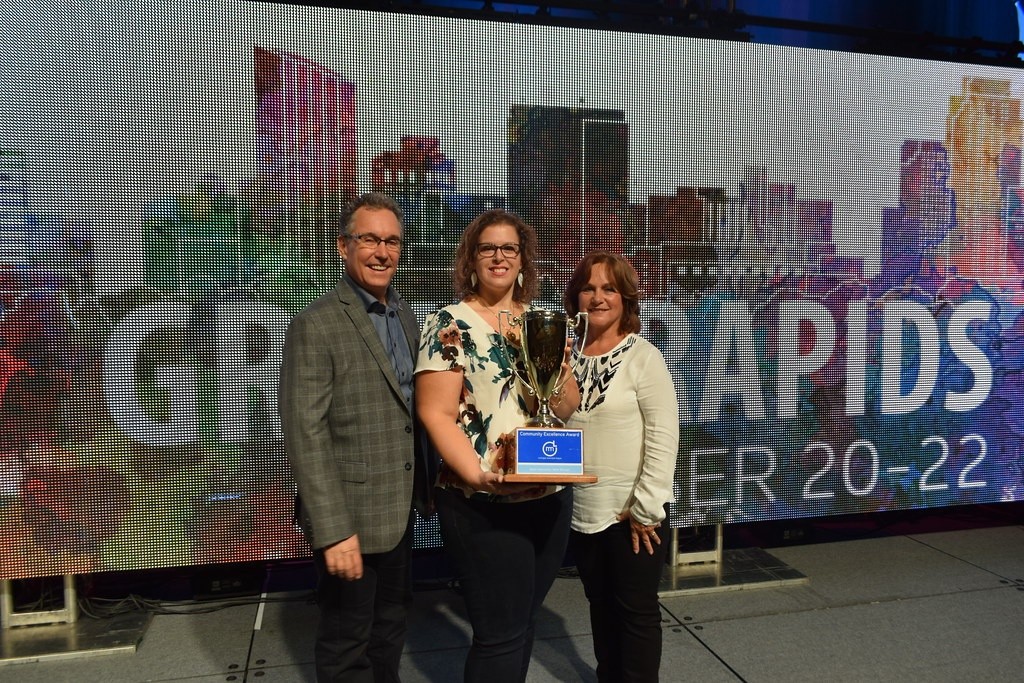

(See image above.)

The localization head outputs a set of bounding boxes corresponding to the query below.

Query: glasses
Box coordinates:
[477,243,522,259]
[345,233,404,250]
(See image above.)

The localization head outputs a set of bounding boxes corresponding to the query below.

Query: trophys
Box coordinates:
[498,310,599,482]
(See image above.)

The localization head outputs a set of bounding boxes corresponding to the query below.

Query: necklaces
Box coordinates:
[478,296,515,341]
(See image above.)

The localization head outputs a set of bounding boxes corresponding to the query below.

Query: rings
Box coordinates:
[651,531,655,537]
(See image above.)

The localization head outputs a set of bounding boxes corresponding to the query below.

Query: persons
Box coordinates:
[413,210,581,683]
[565,250,680,683]
[279,194,440,683]
